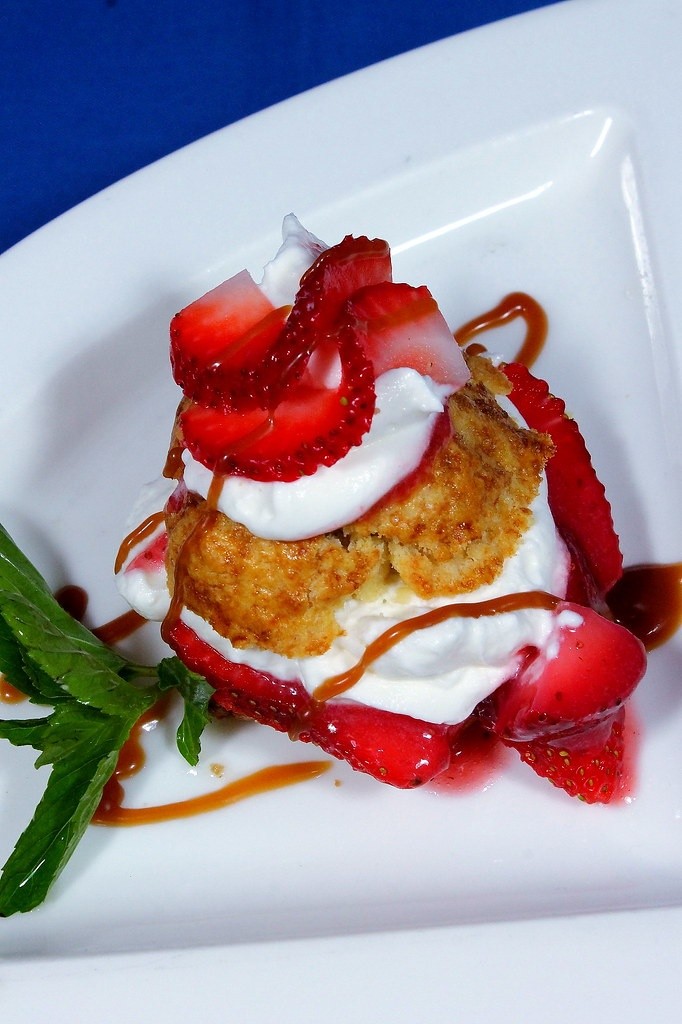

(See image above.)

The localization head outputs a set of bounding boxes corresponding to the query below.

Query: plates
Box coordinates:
[0,0,682,1024]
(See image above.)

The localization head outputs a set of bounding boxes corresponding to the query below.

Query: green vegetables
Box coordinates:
[0,521,217,921]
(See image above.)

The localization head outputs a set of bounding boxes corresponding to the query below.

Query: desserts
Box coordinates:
[109,213,648,807]
